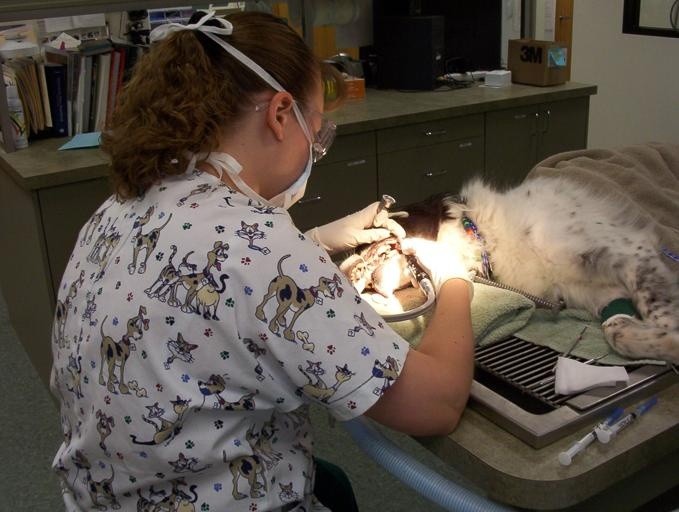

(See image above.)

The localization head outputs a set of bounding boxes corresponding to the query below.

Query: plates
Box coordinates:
[0,73,600,414]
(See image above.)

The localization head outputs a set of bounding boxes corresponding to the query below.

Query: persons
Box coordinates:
[46,10,474,511]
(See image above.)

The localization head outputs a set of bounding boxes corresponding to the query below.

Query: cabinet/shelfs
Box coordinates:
[6,85,30,150]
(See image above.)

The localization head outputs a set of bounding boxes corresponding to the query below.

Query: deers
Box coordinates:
[239,101,315,212]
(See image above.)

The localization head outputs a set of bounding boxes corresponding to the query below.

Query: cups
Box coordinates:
[254,98,338,164]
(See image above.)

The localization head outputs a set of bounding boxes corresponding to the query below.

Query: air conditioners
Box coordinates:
[336,173,679,370]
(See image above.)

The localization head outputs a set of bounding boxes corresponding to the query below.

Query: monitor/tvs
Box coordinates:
[0,37,128,150]
